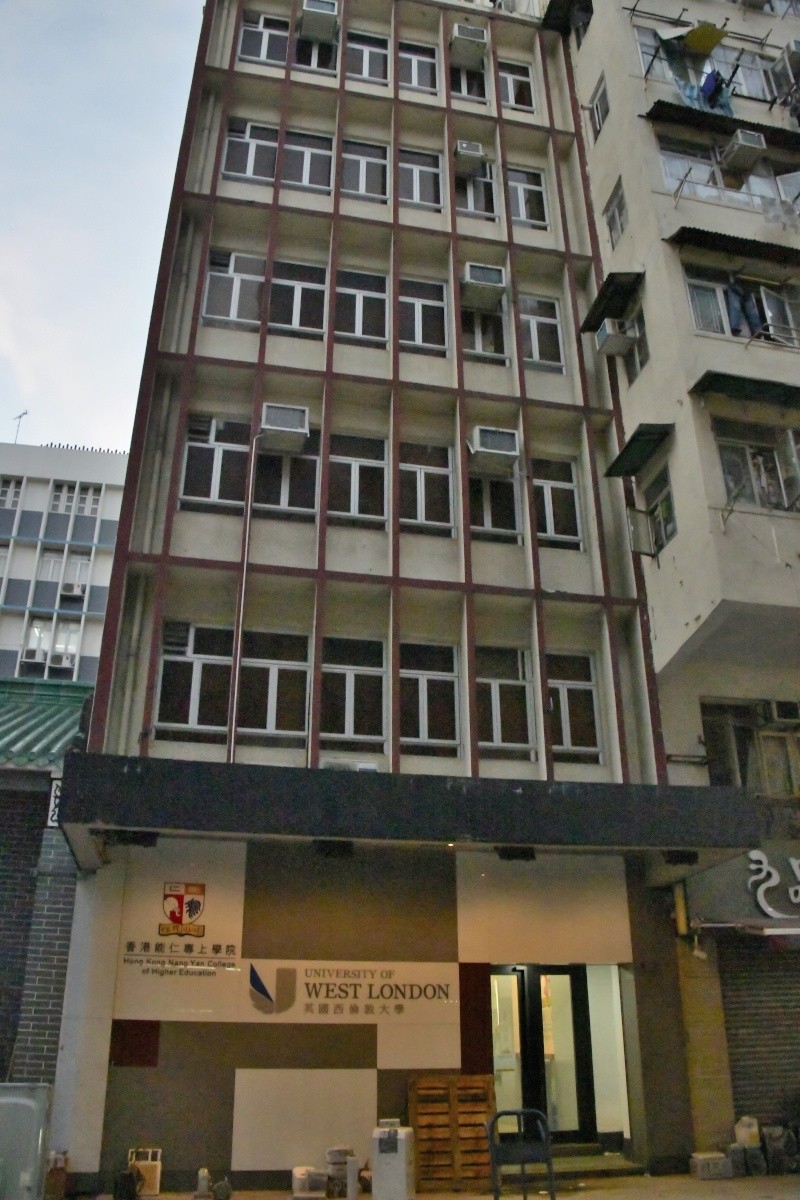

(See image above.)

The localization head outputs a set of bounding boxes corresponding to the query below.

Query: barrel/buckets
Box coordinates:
[735,1116,760,1148]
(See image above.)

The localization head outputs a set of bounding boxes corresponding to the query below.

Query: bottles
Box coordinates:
[198,1168,209,1193]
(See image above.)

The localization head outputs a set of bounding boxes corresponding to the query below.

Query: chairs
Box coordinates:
[482,1107,558,1200]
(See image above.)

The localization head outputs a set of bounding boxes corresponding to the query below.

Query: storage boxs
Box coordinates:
[689,1152,732,1180]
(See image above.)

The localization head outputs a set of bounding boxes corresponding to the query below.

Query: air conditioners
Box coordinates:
[257,401,310,453]
[725,129,765,170]
[468,425,521,476]
[595,318,635,354]
[454,140,486,167]
[449,23,488,65]
[302,0,338,42]
[460,262,506,309]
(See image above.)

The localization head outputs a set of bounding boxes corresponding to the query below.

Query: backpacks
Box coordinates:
[112,1171,137,1200]
